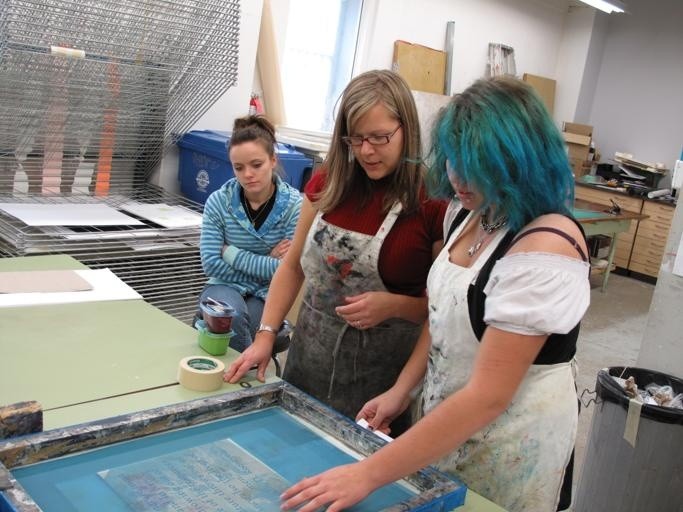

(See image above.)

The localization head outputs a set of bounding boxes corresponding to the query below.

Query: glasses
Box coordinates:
[341,125,400,146]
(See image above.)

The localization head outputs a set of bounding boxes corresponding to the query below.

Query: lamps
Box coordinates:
[578,0,628,15]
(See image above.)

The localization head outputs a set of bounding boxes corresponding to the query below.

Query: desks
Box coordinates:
[568,197,649,294]
[0,254,510,512]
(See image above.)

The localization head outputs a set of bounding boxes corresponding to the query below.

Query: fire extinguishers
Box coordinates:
[249,92,259,117]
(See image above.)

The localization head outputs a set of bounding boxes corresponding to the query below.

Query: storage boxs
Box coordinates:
[587,234,611,260]
[175,130,314,203]
[561,122,601,176]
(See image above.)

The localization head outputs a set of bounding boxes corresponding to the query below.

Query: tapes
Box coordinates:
[178,356,225,392]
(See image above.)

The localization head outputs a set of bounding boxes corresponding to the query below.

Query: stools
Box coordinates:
[192,307,293,377]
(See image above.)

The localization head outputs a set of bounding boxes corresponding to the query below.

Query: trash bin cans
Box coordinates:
[571,366,683,512]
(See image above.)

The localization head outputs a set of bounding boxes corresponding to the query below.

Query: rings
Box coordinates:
[356,320,360,327]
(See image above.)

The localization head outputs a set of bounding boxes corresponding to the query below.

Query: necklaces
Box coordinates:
[467,212,506,257]
[242,191,273,228]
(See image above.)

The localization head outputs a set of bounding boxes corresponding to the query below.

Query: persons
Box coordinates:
[280,76,591,512]
[192,115,305,353]
[222,70,451,441]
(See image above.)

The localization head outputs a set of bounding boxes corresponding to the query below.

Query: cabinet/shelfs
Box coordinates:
[573,183,644,286]
[628,199,677,278]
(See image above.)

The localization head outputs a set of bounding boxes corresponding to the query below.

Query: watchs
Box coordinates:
[255,324,276,333]
